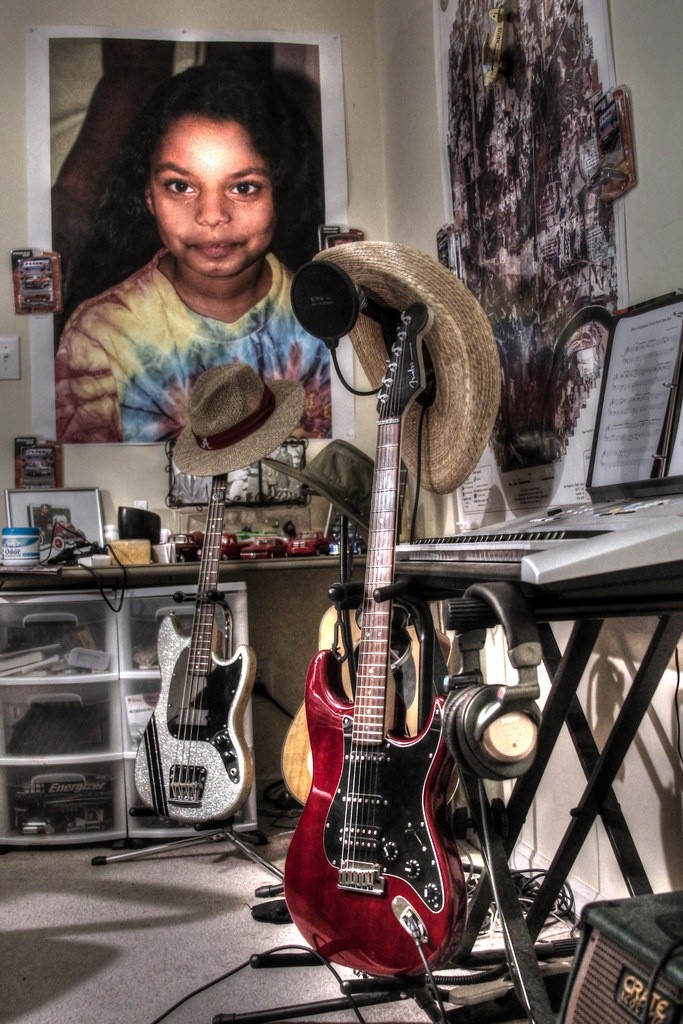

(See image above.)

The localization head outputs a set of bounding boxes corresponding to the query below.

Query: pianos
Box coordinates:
[392,494,682,586]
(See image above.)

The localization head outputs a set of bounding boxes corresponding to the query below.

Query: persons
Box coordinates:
[37,504,53,526]
[55,64,332,443]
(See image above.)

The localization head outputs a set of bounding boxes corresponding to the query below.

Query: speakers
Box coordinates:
[554,890,683,1024]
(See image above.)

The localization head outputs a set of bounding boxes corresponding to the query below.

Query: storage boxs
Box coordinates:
[108,539,151,565]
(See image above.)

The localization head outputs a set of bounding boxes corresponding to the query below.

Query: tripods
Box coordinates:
[91,580,452,1024]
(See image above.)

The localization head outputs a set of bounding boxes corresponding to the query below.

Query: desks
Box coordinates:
[1,551,367,578]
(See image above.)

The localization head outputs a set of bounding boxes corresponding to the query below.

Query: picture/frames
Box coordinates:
[13,436,63,489]
[4,487,105,566]
[164,437,311,509]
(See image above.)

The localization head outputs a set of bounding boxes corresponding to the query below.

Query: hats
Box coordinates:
[311,241,502,495]
[172,362,305,477]
[259,439,374,544]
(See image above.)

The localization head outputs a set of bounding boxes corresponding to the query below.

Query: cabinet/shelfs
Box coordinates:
[0,581,269,848]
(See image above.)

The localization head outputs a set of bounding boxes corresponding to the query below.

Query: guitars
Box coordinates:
[279,299,473,983]
[131,470,261,828]
[276,457,452,805]
